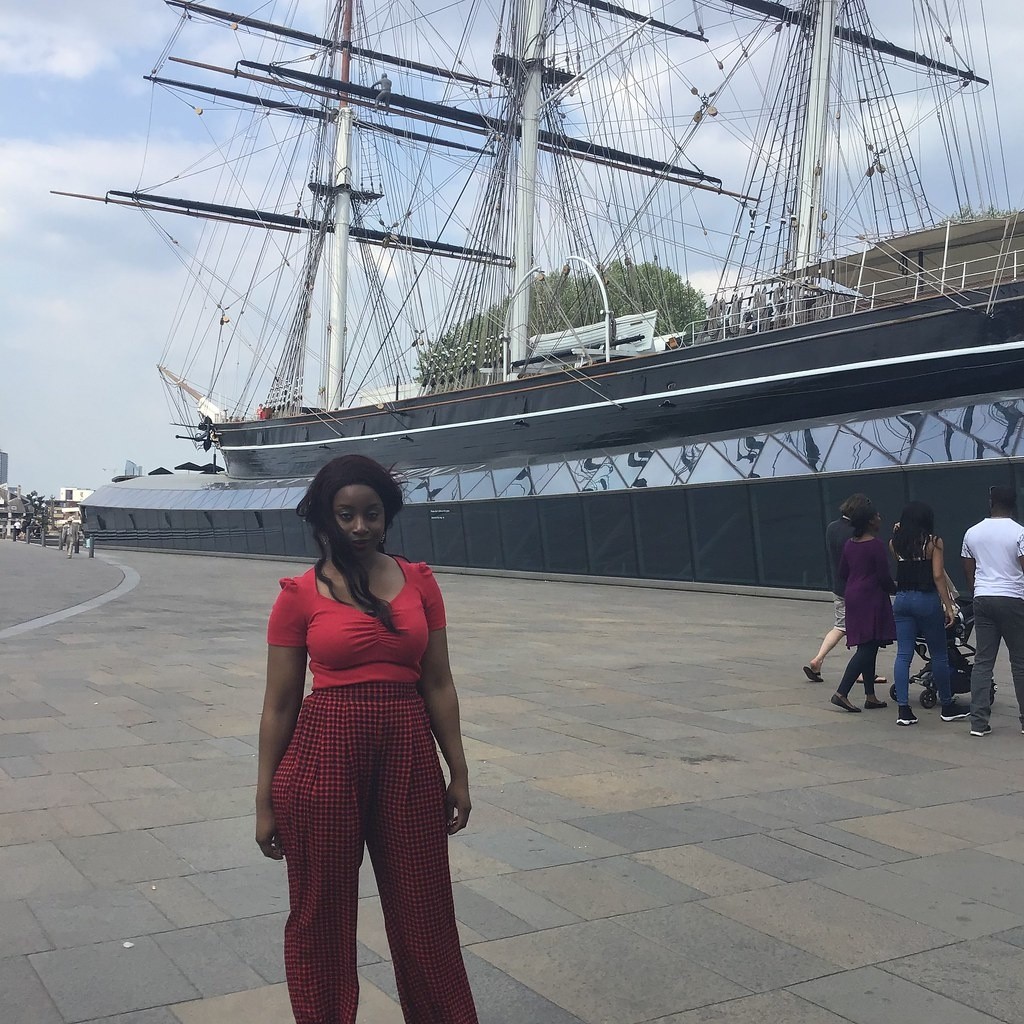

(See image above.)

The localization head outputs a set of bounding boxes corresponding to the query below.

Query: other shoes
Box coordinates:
[831,695,861,712]
[864,700,887,708]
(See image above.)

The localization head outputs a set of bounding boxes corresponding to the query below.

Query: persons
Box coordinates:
[255,403,272,420]
[830,502,897,713]
[61,518,78,559]
[804,495,888,683]
[960,485,1024,737]
[14,519,22,540]
[889,501,971,726]
[256,455,479,1024]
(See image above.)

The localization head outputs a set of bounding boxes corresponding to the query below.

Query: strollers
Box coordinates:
[890,598,998,707]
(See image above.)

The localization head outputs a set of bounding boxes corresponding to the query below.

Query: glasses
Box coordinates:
[988,486,996,508]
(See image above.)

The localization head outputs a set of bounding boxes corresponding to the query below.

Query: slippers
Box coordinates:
[857,674,887,683]
[803,666,823,681]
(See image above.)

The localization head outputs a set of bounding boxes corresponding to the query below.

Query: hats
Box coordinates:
[68,518,72,521]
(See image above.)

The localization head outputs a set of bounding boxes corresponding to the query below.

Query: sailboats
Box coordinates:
[48,1,1024,476]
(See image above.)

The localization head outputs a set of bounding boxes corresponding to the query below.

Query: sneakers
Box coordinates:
[970,725,992,737]
[1022,729,1024,734]
[941,701,971,721]
[897,706,918,726]
